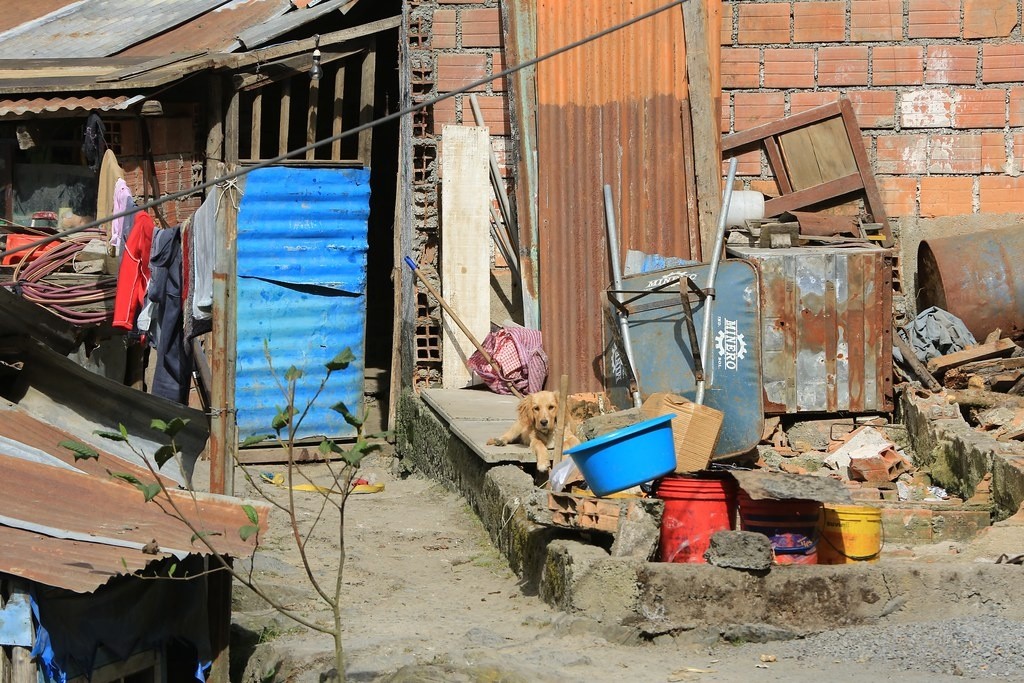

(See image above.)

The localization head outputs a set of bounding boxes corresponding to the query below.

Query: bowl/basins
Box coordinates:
[562,412,679,498]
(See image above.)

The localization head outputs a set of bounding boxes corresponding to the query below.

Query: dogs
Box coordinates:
[485,390,580,473]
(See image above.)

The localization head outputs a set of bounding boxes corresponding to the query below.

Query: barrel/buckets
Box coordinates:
[649,471,738,567]
[740,487,821,563]
[815,502,885,566]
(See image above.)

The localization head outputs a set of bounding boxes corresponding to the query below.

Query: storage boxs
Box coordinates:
[546,223,1023,565]
